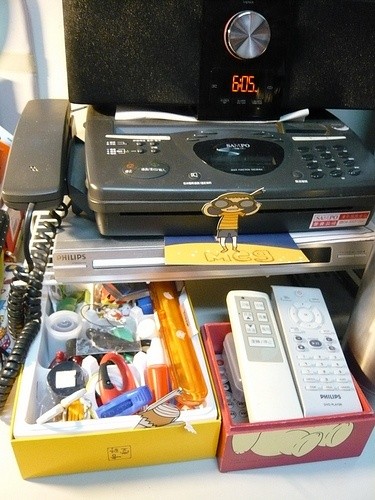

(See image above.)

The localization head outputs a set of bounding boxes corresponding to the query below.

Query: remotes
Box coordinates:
[226,285,362,421]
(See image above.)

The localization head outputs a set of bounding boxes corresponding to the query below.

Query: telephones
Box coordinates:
[2,98,375,235]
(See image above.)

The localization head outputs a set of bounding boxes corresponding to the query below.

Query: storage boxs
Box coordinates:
[6,280,224,480]
[199,322,375,475]
